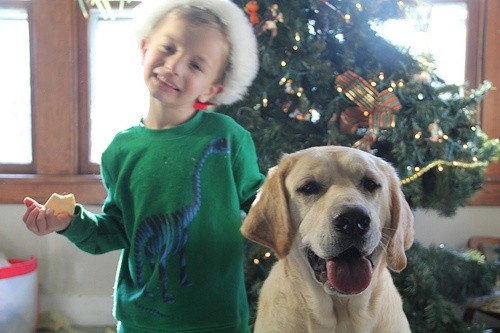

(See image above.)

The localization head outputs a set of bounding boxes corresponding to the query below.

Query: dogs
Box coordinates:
[237,144,416,333]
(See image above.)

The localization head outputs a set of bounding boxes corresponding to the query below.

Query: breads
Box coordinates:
[44,192,76,216]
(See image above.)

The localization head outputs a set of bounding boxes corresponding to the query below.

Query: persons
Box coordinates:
[23,0,266,333]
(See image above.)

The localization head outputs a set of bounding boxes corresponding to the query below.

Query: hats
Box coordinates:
[128,1,261,105]
